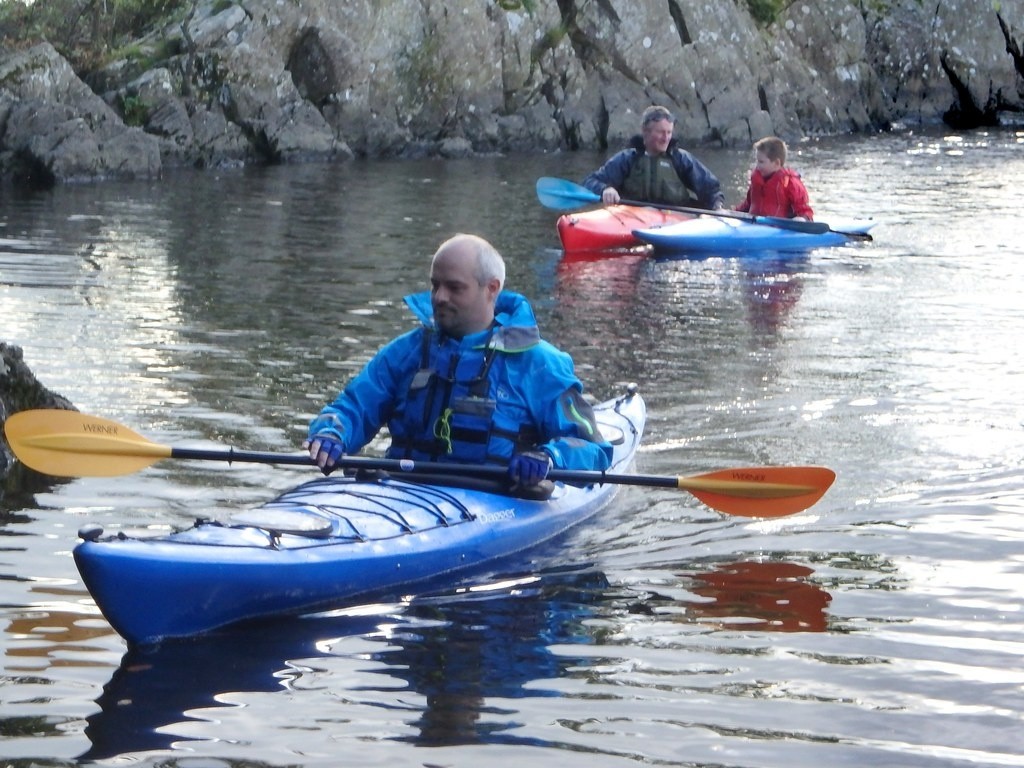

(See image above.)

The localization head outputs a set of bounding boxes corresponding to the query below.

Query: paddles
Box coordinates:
[539,174,829,236]
[3,406,838,520]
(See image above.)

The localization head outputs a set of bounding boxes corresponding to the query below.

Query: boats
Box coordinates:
[630,208,879,262]
[552,204,700,262]
[70,383,654,660]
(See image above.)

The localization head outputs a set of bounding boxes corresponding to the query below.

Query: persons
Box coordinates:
[717,137,814,222]
[370,562,609,746]
[742,249,810,347]
[300,234,614,491]
[583,106,726,211]
